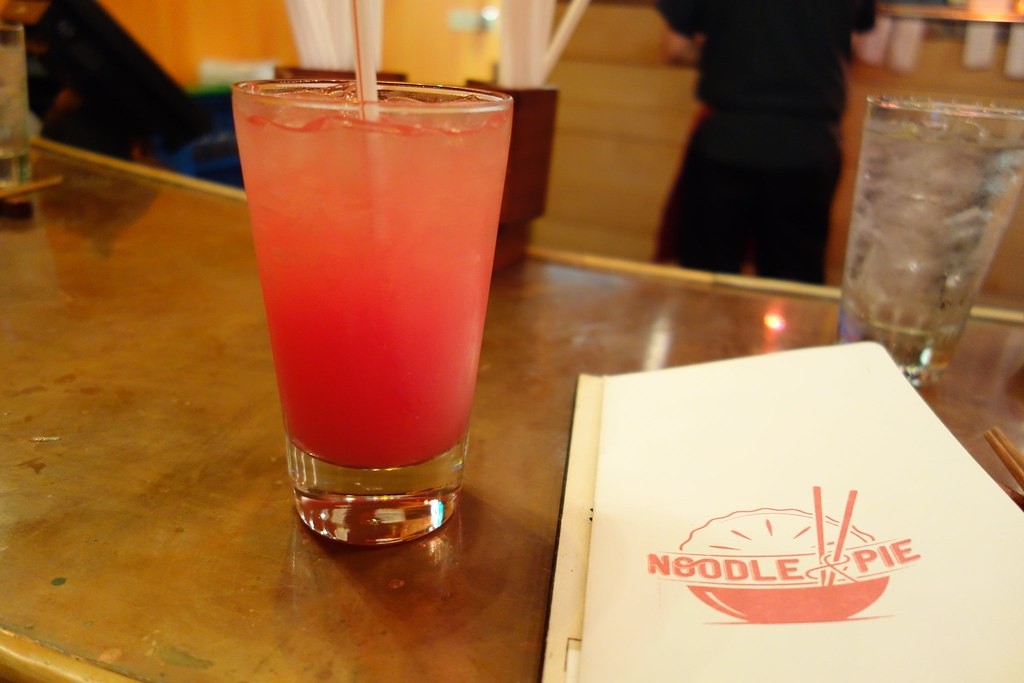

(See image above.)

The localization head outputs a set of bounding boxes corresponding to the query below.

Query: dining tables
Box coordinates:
[1,133,1023,683]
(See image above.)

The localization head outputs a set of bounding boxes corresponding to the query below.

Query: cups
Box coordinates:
[0,21,34,185]
[464,80,560,222]
[230,77,513,545]
[275,64,410,83]
[833,95,1022,391]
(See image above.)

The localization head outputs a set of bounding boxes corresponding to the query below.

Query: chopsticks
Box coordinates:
[983,425,1024,492]
